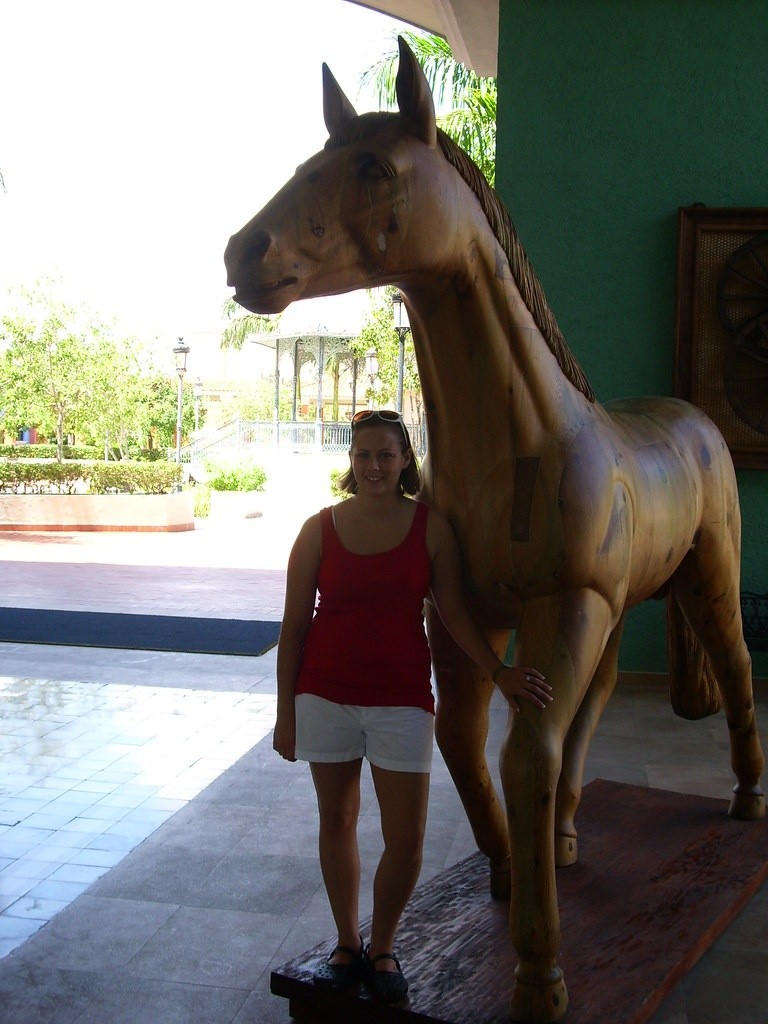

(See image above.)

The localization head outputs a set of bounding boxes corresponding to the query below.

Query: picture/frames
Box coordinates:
[673,206,768,471]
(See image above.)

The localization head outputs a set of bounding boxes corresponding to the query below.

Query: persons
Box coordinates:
[272,410,556,998]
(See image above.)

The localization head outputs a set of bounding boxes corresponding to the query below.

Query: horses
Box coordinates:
[223,28,768,1024]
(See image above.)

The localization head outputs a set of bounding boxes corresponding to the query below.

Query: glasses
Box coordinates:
[351,410,408,446]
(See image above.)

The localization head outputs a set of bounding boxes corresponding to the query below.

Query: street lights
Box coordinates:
[171,334,190,494]
[391,289,412,415]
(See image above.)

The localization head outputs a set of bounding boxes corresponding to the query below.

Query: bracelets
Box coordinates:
[492,664,507,682]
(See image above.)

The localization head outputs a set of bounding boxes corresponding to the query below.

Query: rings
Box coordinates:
[525,675,530,682]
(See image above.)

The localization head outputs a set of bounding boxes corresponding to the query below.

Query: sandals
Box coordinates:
[362,943,408,1003]
[313,935,364,985]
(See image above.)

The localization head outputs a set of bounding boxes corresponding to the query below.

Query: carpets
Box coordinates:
[0,607,281,657]
